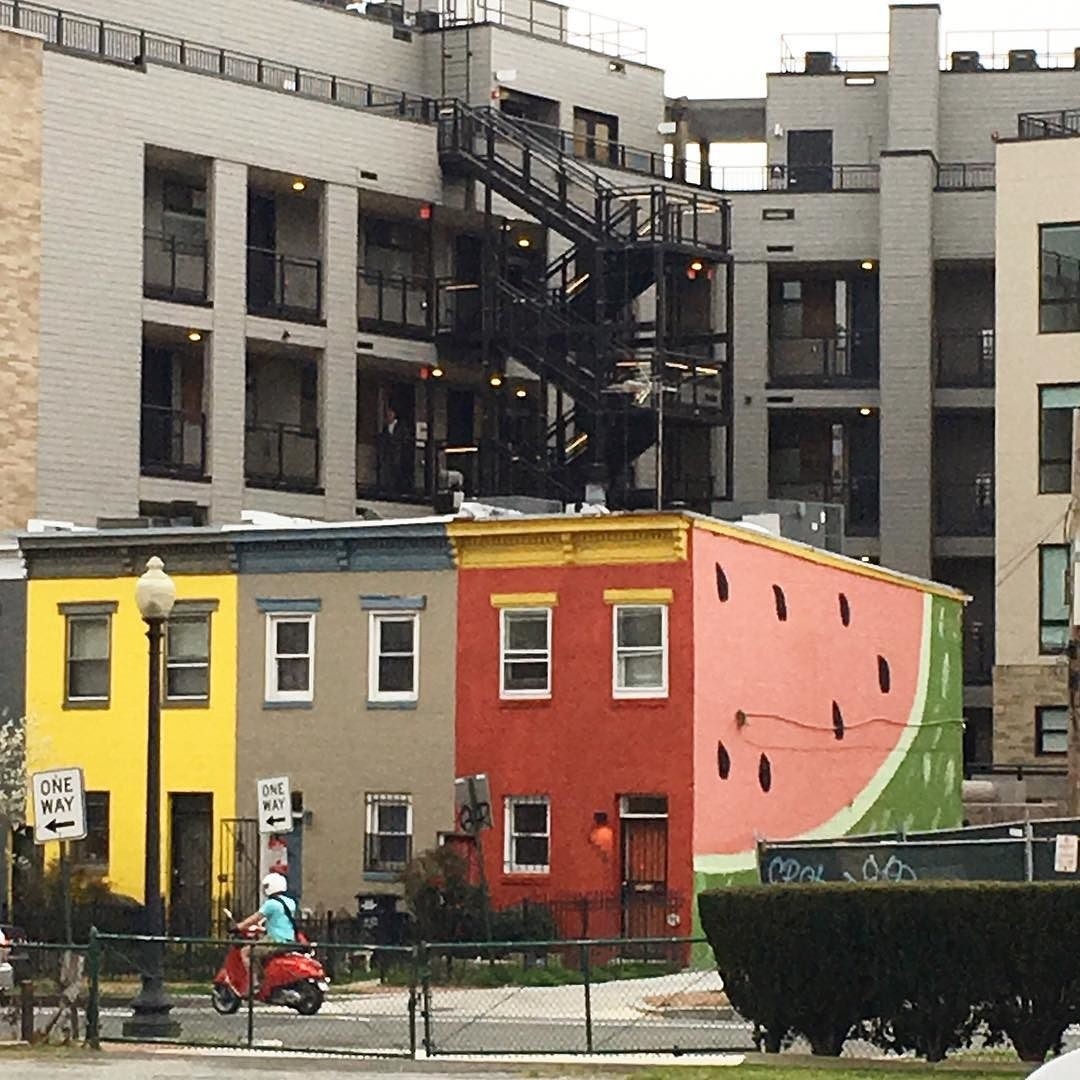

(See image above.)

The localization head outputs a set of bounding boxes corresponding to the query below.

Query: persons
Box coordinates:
[235,872,298,995]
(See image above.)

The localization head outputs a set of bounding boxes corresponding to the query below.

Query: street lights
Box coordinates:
[123,555,183,1039]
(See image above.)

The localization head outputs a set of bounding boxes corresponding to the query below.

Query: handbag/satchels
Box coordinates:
[296,931,311,946]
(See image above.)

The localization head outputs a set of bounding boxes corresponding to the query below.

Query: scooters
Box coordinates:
[210,907,332,1016]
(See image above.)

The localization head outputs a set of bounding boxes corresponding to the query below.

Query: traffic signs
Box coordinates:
[257,776,293,832]
[33,767,85,842]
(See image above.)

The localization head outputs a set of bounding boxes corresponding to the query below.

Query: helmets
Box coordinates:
[262,873,287,897]
